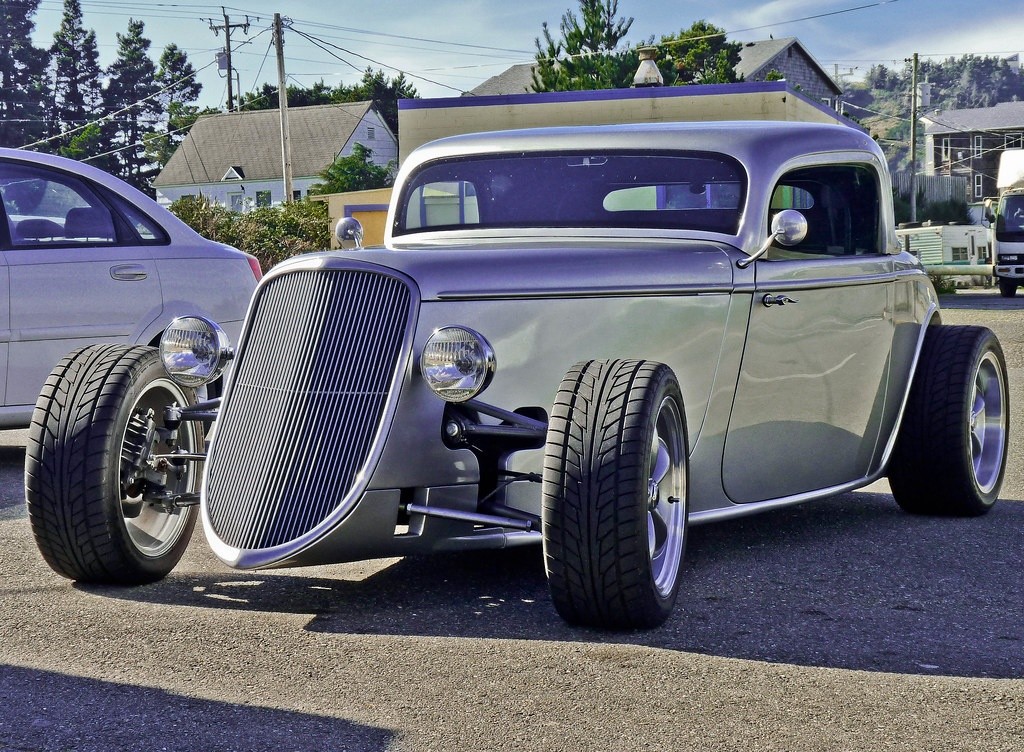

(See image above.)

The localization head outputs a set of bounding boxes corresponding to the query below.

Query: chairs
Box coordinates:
[16,218,64,242]
[64,207,112,241]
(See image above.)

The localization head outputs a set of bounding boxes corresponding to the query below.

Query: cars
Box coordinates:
[0,146,264,434]
[23,115,1011,634]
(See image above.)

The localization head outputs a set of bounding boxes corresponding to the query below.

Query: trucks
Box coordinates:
[980,149,1024,297]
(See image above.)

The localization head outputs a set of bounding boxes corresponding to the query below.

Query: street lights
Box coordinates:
[909,106,943,223]
